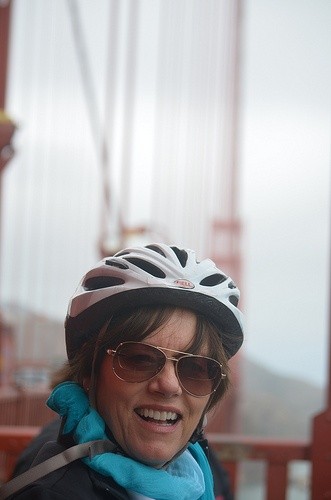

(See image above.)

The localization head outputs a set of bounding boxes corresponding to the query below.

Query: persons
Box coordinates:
[5,242,252,500]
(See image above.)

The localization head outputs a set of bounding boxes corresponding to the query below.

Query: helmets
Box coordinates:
[64,243,245,376]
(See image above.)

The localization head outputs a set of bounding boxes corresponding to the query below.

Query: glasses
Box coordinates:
[105,341,227,398]
